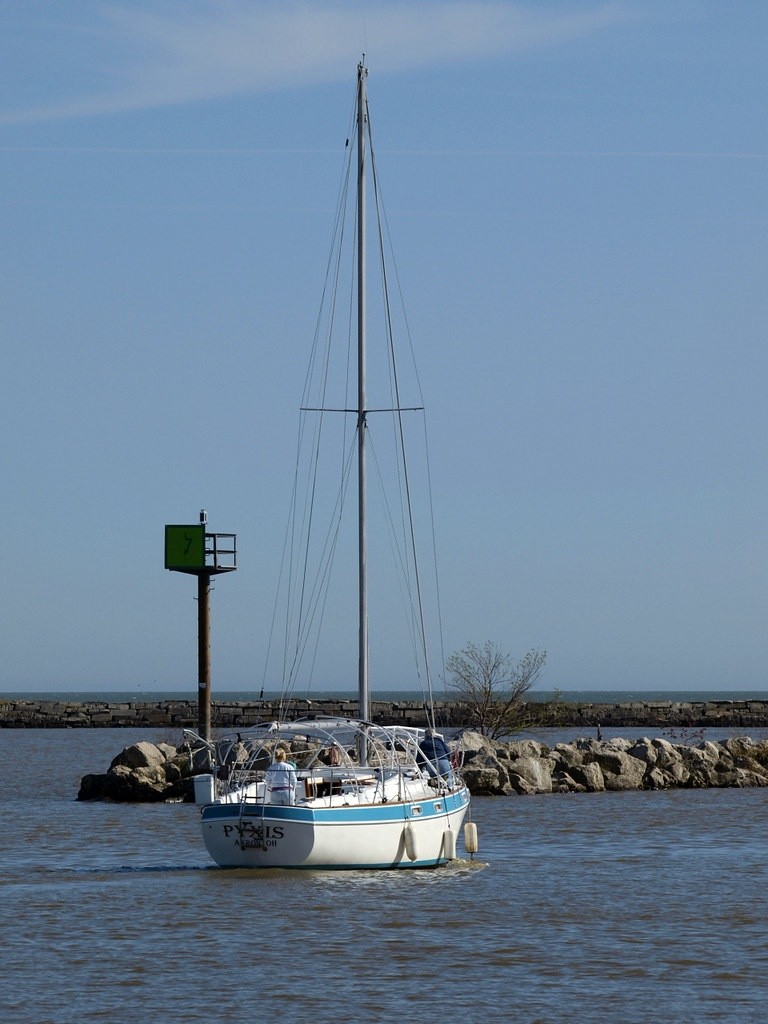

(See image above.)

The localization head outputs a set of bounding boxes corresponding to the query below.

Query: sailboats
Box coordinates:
[191,52,471,873]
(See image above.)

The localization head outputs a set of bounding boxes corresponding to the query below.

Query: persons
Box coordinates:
[416,729,453,792]
[262,748,297,806]
